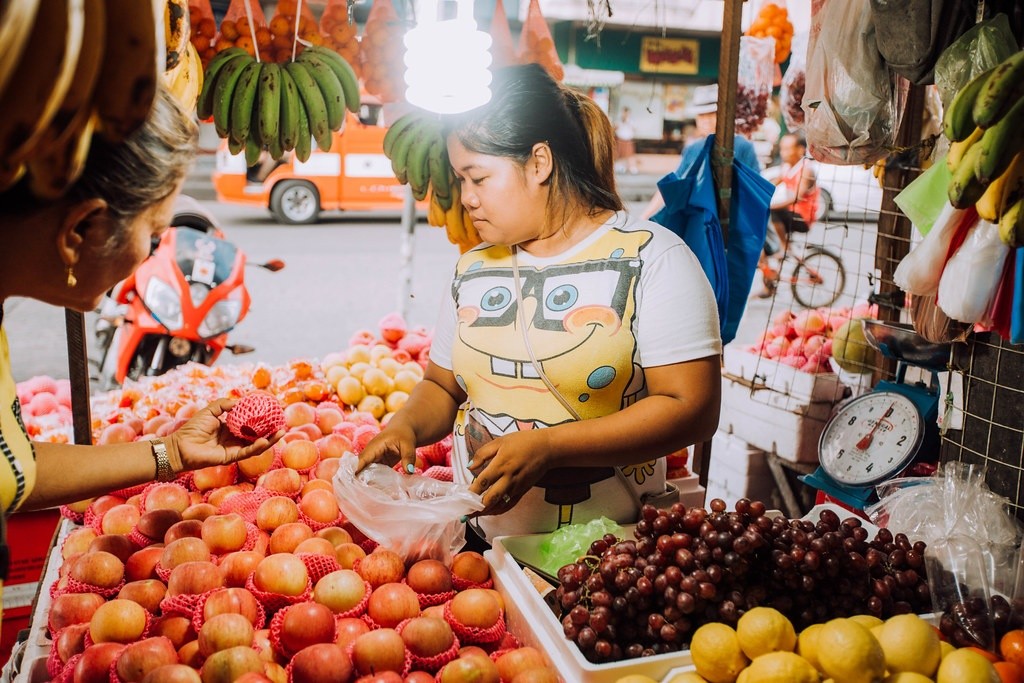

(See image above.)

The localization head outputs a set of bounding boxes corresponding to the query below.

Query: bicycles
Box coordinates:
[766,221,847,310]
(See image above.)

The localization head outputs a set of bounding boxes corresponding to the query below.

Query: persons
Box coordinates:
[0,0,285,531]
[354,64,723,551]
[759,134,821,298]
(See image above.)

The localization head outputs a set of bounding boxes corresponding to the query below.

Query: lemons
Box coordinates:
[615,607,1003,683]
[321,343,424,424]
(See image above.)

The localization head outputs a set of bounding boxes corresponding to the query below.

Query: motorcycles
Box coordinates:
[87,193,284,391]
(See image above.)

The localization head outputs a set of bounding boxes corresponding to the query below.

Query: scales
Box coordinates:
[798,318,972,511]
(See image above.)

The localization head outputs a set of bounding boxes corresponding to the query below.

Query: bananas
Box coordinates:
[383,111,484,253]
[944,51,1024,248]
[0,0,204,198]
[199,46,360,167]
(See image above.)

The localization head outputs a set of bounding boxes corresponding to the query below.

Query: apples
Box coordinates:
[747,291,912,374]
[16,359,559,683]
[352,317,433,371]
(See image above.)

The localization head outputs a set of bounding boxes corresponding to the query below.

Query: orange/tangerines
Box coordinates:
[745,3,794,62]
[187,0,564,104]
[930,625,1024,683]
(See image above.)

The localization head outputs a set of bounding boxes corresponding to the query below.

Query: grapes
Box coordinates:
[559,499,1024,657]
[736,70,805,137]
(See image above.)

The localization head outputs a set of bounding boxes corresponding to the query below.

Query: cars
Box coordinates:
[209,111,432,226]
[804,158,882,222]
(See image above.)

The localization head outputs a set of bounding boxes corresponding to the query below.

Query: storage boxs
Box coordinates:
[12,349,989,683]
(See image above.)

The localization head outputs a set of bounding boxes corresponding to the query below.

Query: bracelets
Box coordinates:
[150,438,177,483]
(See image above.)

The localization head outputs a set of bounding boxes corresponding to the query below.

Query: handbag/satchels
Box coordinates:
[329,450,487,561]
[800,1,894,166]
[634,480,681,520]
